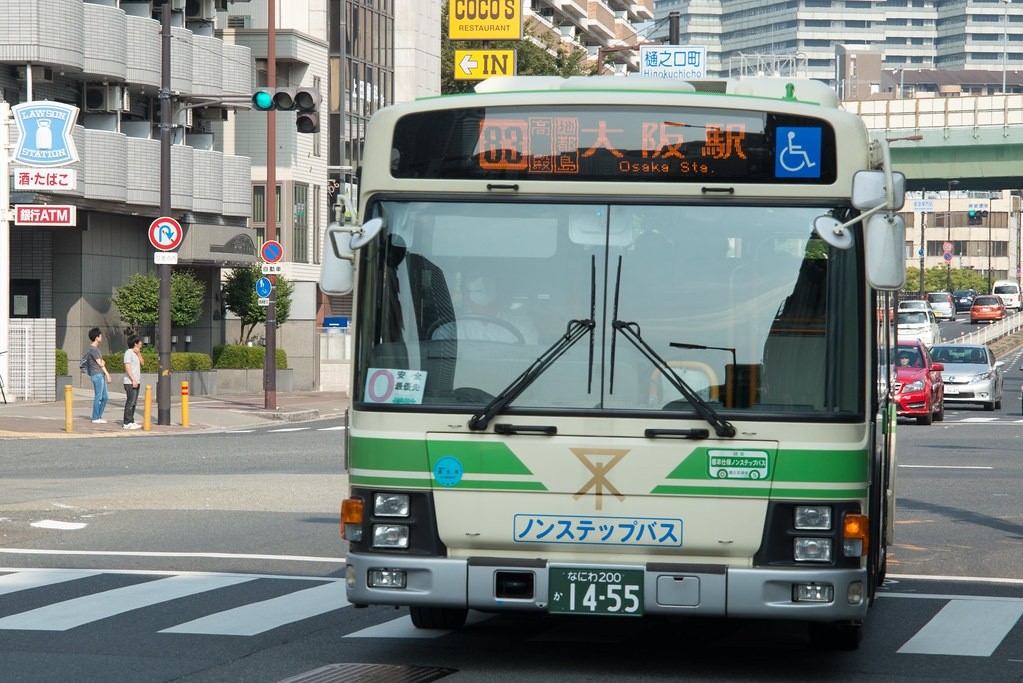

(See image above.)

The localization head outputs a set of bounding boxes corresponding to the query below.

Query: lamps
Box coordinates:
[217,289,226,300]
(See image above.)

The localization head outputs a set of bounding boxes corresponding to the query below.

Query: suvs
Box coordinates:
[895,338,944,426]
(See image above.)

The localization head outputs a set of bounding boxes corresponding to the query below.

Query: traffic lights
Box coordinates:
[967,211,988,225]
[251,86,324,133]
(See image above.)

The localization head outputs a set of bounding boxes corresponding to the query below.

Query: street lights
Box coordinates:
[947,181,960,292]
[988,197,999,295]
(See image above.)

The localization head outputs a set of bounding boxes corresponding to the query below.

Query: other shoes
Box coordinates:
[133,423,142,428]
[123,423,137,429]
[92,419,107,424]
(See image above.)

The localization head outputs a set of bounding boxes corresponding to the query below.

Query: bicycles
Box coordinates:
[0,351,8,404]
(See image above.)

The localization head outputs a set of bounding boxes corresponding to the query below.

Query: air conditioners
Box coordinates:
[172,102,193,127]
[152,0,214,22]
[82,86,131,112]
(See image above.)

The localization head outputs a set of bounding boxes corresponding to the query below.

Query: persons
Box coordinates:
[431,263,539,345]
[87,328,112,425]
[897,351,911,367]
[123,335,145,430]
[971,351,982,361]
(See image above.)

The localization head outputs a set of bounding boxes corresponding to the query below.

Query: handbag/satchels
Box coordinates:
[79,353,88,373]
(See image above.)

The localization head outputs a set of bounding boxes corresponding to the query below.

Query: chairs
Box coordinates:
[940,349,952,362]
[970,350,983,363]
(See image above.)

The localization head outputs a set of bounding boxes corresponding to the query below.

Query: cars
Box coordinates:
[952,290,978,312]
[927,344,1003,411]
[970,295,1008,324]
[1020,365,1023,415]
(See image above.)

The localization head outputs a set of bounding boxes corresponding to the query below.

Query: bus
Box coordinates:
[329,74,906,648]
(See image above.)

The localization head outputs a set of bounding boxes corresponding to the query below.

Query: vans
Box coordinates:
[926,292,956,321]
[897,300,933,322]
[992,280,1023,311]
[896,308,942,346]
